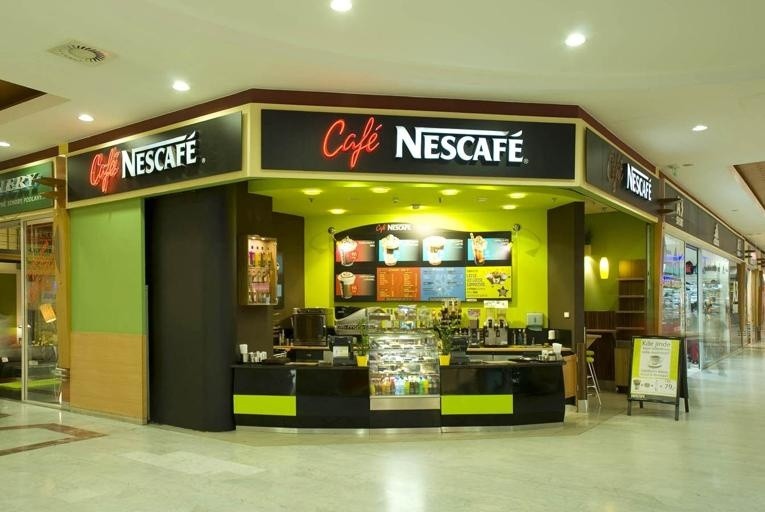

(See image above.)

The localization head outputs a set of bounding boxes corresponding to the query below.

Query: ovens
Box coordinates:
[290,307,335,347]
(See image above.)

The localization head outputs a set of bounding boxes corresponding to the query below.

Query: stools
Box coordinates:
[586,350,602,404]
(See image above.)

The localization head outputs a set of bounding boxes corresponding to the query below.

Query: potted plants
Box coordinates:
[355,318,372,367]
[437,320,458,367]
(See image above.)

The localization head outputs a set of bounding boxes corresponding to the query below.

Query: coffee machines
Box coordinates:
[484,300,509,347]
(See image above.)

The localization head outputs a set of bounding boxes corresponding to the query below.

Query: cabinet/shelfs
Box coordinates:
[368,333,441,410]
[238,233,278,306]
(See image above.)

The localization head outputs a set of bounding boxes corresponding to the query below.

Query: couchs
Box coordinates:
[0,344,57,378]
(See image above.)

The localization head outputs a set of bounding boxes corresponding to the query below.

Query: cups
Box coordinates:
[472,239,486,266]
[382,238,400,266]
[552,343,562,362]
[427,237,445,265]
[337,240,356,299]
[240,344,267,364]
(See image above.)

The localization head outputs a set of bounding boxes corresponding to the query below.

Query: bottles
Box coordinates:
[248,245,272,304]
[512,328,527,345]
[387,308,395,328]
[371,375,439,395]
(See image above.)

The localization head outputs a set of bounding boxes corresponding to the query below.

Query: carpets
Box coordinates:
[0,377,63,391]
